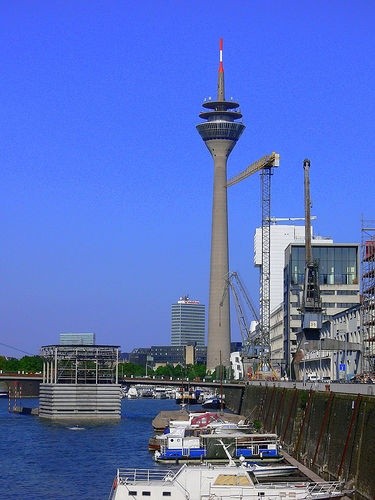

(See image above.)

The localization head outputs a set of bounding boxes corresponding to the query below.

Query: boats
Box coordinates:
[248,465,299,478]
[120,384,226,408]
[152,433,287,465]
[109,438,345,500]
[148,412,258,451]
[151,410,255,432]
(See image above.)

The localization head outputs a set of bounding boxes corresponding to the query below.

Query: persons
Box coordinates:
[345,359,351,383]
[340,361,345,380]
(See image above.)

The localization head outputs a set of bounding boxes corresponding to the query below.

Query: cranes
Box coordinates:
[218,151,281,380]
